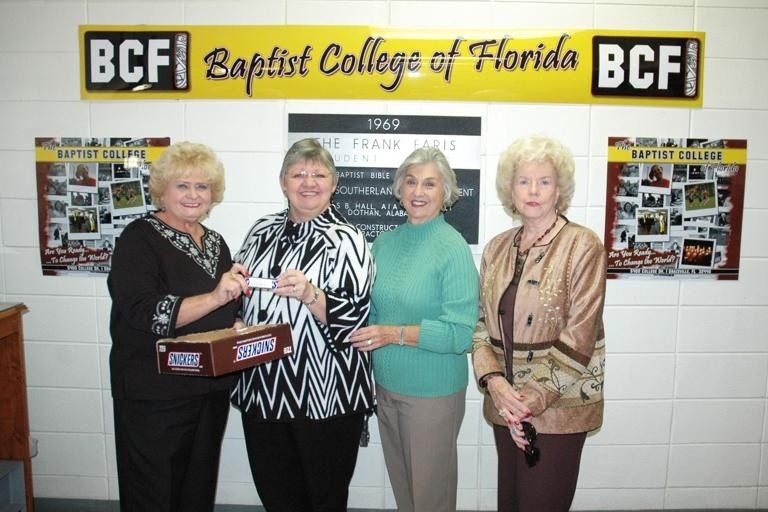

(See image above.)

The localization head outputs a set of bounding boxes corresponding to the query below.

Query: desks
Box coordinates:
[0,297,36,512]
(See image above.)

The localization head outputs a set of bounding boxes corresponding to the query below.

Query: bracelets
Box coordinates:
[305,284,319,306]
[399,326,406,346]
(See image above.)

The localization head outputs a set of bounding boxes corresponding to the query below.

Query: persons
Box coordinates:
[106,141,249,512]
[40,138,158,253]
[469,135,606,512]
[614,137,732,267]
[230,138,375,512]
[347,146,480,512]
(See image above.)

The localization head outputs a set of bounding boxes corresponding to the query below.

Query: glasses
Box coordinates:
[286,166,336,182]
[519,419,540,470]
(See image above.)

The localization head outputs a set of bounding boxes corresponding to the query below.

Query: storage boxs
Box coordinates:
[156,322,294,379]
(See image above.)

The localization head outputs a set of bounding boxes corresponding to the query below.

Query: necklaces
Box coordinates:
[515,213,559,256]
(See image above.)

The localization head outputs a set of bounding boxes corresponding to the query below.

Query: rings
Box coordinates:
[498,408,506,417]
[231,272,236,279]
[367,339,373,346]
[512,429,516,434]
